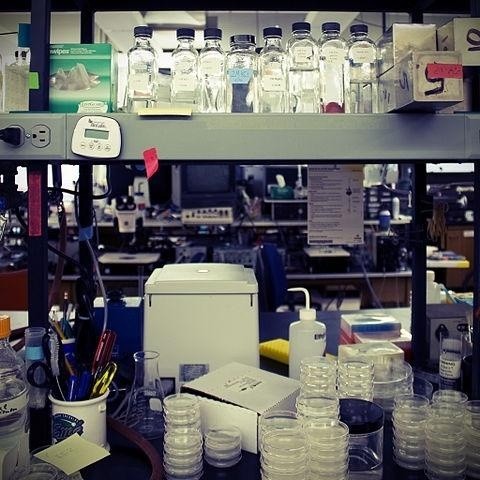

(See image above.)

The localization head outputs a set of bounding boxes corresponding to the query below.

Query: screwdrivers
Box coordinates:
[66,375,77,401]
[90,329,117,380]
[89,360,117,399]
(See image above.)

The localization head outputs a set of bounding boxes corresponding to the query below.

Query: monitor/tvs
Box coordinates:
[172,163,238,207]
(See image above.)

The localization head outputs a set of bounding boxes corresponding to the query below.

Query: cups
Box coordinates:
[48,386,110,451]
[10,463,58,480]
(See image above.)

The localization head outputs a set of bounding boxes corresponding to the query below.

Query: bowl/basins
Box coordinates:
[164,393,243,478]
[257,357,375,480]
[376,359,479,480]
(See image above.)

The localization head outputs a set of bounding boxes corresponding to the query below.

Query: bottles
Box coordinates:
[126,21,378,113]
[439,338,463,382]
[123,351,165,440]
[23,326,47,408]
[425,270,441,304]
[379,210,391,231]
[0,316,33,480]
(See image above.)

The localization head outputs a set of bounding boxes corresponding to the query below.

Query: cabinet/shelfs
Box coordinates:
[0,0,478,479]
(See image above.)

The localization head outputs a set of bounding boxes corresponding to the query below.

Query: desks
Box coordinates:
[48,264,413,310]
[140,208,410,226]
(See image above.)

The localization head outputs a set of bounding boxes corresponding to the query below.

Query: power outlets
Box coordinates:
[0,113,67,158]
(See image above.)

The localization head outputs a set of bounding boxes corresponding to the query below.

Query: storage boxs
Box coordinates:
[50,44,118,113]
[178,361,301,455]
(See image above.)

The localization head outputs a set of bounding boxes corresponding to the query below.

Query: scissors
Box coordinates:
[26,330,66,401]
